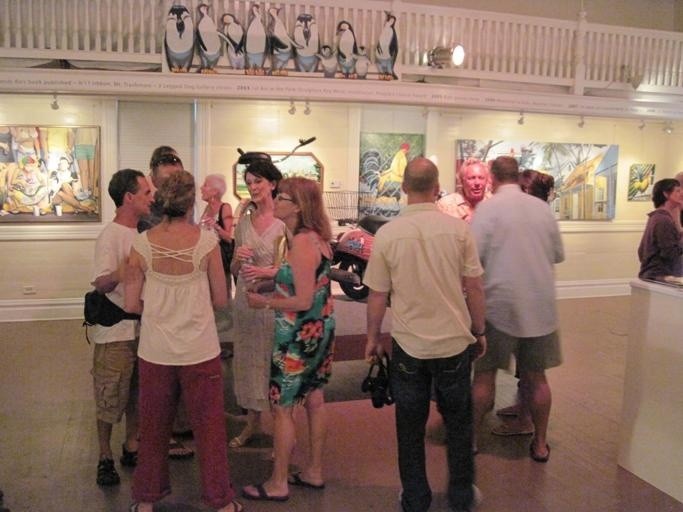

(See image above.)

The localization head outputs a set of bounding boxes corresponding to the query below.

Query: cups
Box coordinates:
[32,206,40,217]
[55,205,63,217]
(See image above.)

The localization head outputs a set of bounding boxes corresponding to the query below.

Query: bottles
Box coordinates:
[199,219,207,231]
[238,246,261,291]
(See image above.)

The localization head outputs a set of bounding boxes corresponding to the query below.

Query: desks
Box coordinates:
[616,281,683,505]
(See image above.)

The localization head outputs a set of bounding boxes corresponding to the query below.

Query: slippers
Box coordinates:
[96,433,325,512]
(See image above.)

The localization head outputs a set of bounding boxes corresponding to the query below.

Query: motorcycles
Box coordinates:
[329,190,447,307]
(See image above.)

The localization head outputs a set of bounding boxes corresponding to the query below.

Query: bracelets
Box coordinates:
[265,299,269,309]
[473,331,486,336]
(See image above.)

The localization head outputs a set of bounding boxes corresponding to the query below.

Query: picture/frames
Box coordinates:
[233,152,324,200]
[0,124,102,223]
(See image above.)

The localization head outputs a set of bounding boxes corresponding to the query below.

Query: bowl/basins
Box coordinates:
[11,211,18,214]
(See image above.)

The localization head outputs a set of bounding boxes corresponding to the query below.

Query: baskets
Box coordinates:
[324,190,377,222]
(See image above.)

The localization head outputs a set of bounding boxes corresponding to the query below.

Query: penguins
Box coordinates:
[164,4,399,81]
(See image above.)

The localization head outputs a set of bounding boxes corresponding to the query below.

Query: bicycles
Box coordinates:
[230,137,318,290]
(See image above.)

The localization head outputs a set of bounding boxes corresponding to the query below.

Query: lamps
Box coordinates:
[304,102,311,115]
[51,94,59,109]
[638,119,674,135]
[518,112,524,124]
[416,44,465,82]
[578,116,585,128]
[288,101,296,115]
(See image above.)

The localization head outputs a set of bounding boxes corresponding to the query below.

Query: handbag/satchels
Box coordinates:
[220,240,235,273]
[84,292,125,326]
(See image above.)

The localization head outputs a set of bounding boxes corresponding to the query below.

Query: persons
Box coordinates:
[364,157,488,511]
[437,156,493,223]
[241,177,333,500]
[490,168,555,437]
[468,156,565,461]
[0,127,99,217]
[83,145,232,487]
[637,178,683,281]
[124,170,244,512]
[230,160,293,462]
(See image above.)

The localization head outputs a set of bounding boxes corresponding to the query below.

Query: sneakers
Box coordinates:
[492,406,550,462]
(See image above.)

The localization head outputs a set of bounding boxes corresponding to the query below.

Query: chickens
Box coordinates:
[359,143,411,201]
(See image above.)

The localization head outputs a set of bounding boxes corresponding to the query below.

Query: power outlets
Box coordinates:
[24,285,37,294]
